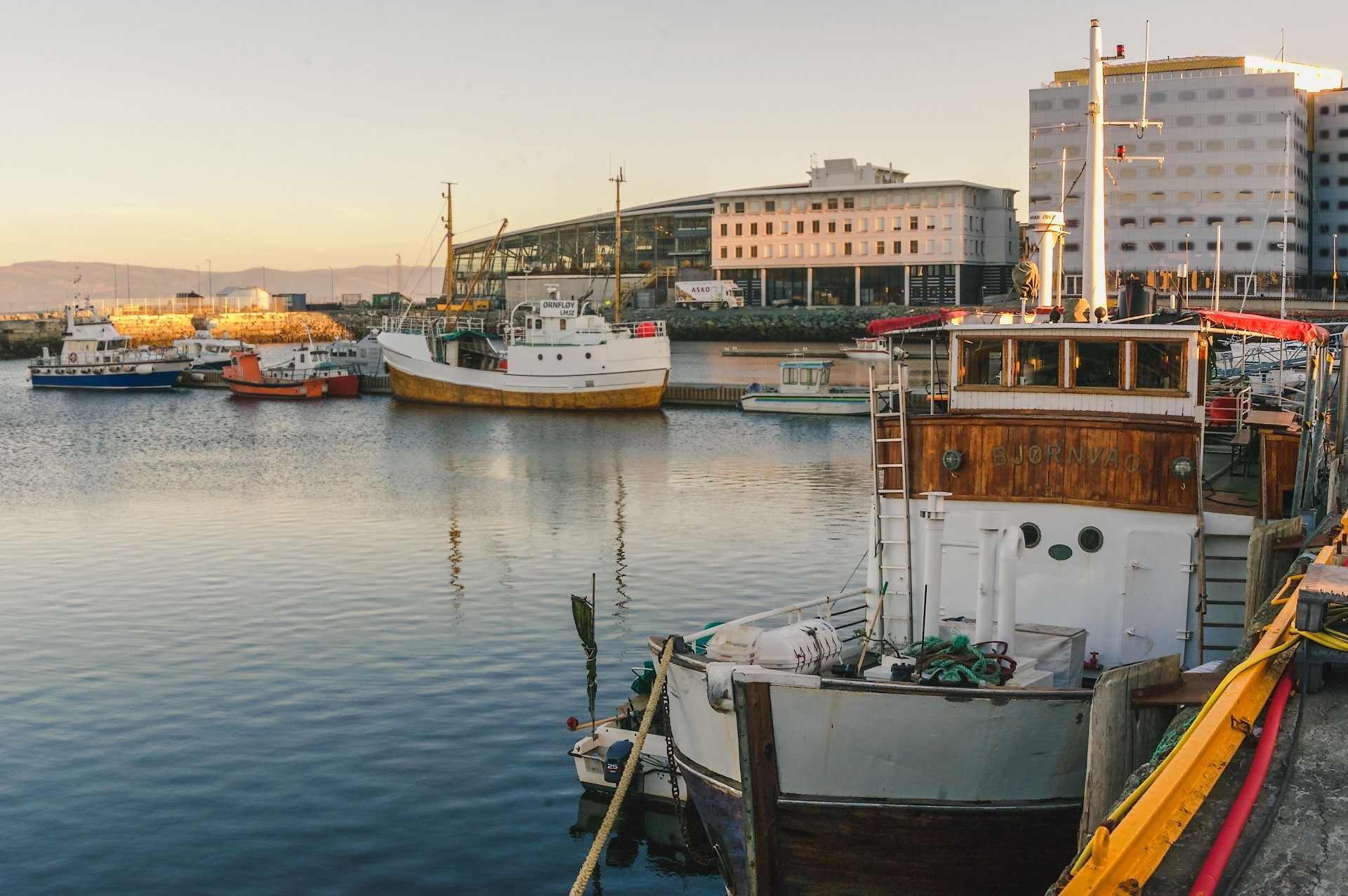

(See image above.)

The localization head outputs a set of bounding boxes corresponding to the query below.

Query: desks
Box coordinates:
[1244,410,1295,477]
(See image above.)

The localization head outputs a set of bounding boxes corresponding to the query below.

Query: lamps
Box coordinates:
[943,450,962,471]
[1171,456,1195,481]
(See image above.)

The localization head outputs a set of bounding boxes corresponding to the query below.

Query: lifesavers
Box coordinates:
[69,352,77,363]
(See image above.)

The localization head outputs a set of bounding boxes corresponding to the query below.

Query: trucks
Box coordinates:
[675,280,744,310]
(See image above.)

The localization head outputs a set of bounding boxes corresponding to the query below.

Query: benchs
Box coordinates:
[1229,429,1257,477]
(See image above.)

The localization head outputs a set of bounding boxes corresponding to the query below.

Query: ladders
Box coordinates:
[868,363,915,655]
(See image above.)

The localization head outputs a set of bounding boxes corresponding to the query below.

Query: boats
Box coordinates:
[739,352,913,416]
[568,705,690,812]
[376,165,672,412]
[838,337,897,360]
[171,312,261,370]
[298,316,487,393]
[647,17,1348,896]
[223,341,365,400]
[27,264,195,391]
